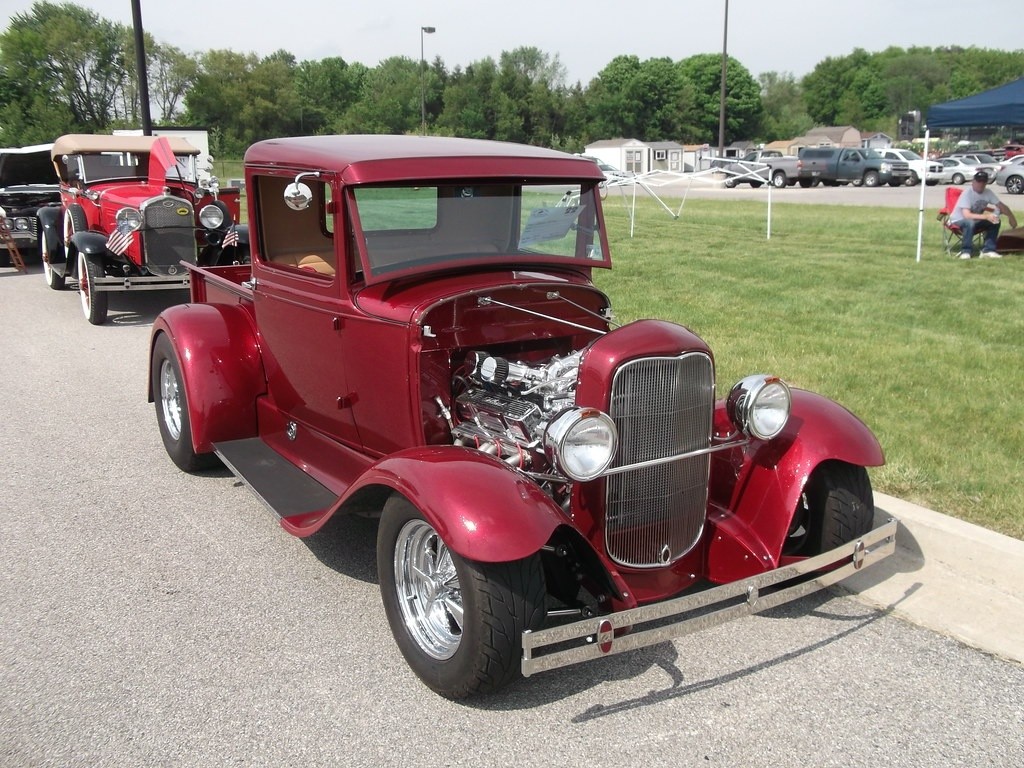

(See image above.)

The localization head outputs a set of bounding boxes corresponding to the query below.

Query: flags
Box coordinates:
[105,221,134,256]
[221,222,240,249]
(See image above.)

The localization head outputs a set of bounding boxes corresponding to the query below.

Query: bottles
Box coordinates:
[993,204,1000,224]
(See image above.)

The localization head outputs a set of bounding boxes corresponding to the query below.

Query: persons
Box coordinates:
[949,171,1017,260]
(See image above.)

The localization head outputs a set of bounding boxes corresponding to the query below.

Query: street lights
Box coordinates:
[419,24,439,136]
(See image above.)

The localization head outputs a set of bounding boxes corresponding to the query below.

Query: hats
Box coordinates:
[975,172,988,182]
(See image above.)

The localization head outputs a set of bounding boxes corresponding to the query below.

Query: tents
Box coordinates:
[916,78,1024,261]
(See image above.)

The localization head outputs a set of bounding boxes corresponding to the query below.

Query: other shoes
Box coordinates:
[961,253,970,259]
[979,251,1002,258]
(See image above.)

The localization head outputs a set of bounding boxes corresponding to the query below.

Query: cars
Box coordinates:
[0,141,123,267]
[932,144,1024,184]
[34,133,251,325]
[994,154,1024,195]
[145,133,902,701]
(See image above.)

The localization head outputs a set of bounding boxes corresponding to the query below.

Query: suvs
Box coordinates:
[865,147,943,187]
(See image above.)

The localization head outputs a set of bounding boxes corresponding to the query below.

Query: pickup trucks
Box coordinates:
[796,144,910,188]
[721,148,798,189]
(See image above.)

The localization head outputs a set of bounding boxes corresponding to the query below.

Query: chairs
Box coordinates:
[936,187,984,256]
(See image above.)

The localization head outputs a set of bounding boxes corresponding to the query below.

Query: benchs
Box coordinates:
[272,242,498,268]
